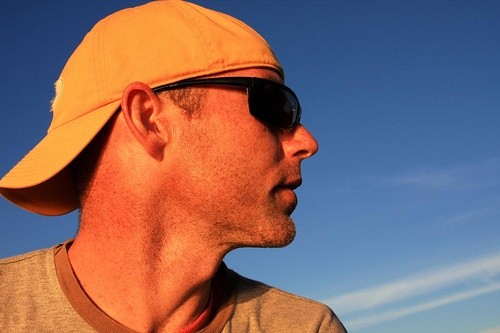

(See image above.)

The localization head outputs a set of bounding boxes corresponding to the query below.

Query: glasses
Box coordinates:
[153,77,302,129]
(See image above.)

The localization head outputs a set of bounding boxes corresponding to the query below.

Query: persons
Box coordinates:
[0,0,348,333]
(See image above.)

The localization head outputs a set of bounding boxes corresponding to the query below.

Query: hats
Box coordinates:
[0,0,284,216]
